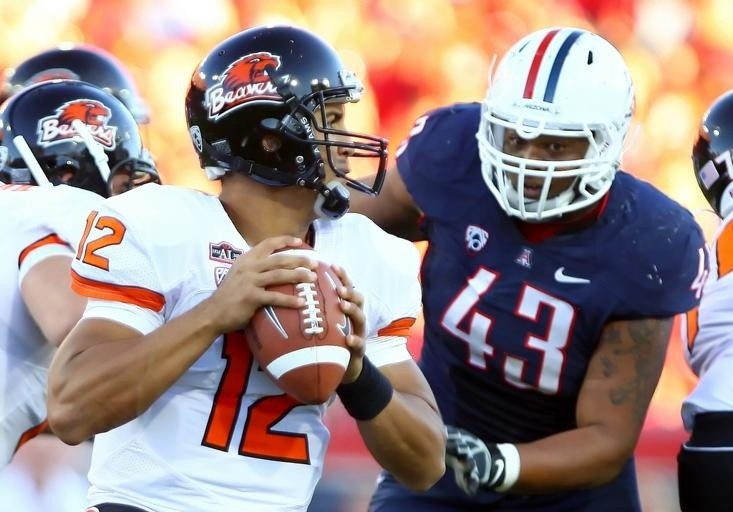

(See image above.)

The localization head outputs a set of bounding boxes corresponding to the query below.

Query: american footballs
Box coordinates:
[245,240,354,405]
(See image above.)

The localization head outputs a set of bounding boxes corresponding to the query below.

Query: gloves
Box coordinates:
[445,427,524,499]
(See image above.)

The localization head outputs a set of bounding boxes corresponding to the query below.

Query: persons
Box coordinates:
[46,25,446,512]
[0,81,145,512]
[676,88,733,510]
[328,26,708,511]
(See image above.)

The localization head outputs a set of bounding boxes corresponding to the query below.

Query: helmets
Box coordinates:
[185,24,389,222]
[690,91,733,218]
[477,28,634,221]
[1,48,161,199]
